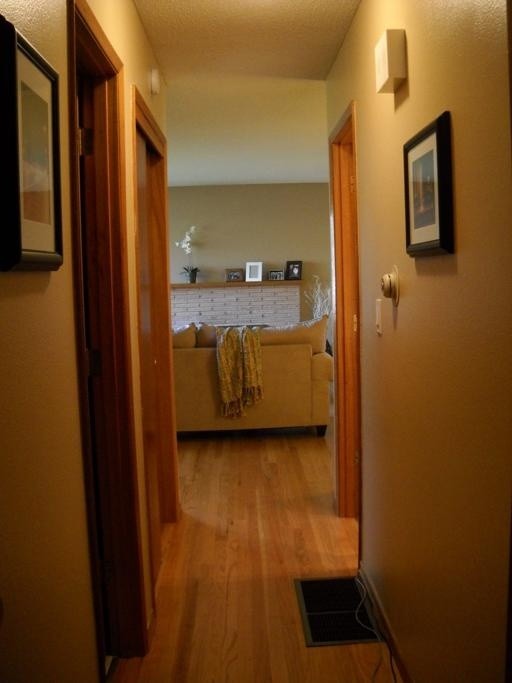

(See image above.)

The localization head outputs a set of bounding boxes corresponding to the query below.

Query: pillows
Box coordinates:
[173,314,329,355]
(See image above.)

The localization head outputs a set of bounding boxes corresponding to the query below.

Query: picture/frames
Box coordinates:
[225,262,301,282]
[403,111,455,257]
[0,14,64,272]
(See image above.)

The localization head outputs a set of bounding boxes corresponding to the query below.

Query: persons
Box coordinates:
[289,267,299,277]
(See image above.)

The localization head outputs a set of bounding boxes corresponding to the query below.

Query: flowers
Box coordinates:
[170,225,200,277]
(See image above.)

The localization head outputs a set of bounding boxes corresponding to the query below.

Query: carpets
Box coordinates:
[293,576,382,647]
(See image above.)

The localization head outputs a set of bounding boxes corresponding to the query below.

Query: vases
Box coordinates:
[190,270,196,282]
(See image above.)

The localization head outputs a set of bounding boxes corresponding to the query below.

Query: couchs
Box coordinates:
[173,343,335,437]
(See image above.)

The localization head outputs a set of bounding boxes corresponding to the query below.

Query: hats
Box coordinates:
[381,264,399,307]
